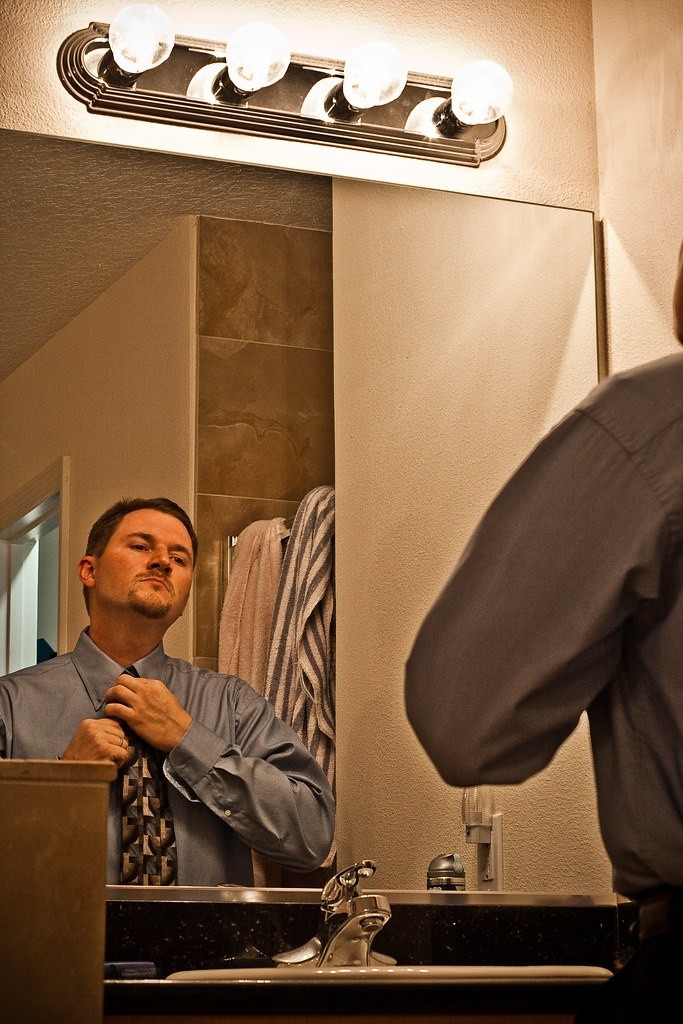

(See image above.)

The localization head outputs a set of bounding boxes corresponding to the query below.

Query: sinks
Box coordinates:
[166,966,612,981]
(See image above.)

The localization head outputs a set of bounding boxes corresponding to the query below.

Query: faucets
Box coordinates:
[271,859,398,968]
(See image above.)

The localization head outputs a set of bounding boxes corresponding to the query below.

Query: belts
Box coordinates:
[614,892,671,950]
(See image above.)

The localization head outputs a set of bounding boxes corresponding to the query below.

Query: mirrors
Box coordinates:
[0,128,621,894]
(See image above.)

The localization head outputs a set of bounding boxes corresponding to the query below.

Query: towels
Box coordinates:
[262,485,339,868]
[218,516,290,699]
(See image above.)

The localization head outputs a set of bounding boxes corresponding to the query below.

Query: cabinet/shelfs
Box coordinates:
[0,757,118,1024]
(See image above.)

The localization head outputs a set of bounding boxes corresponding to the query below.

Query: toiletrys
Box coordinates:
[425,850,466,891]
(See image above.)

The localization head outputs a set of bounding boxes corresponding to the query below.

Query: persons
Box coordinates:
[0,497,334,886]
[406,242,683,1024]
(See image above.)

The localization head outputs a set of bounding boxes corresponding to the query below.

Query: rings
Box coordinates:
[119,738,123,747]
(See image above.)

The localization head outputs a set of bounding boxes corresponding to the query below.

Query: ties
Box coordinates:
[121,719,179,886]
